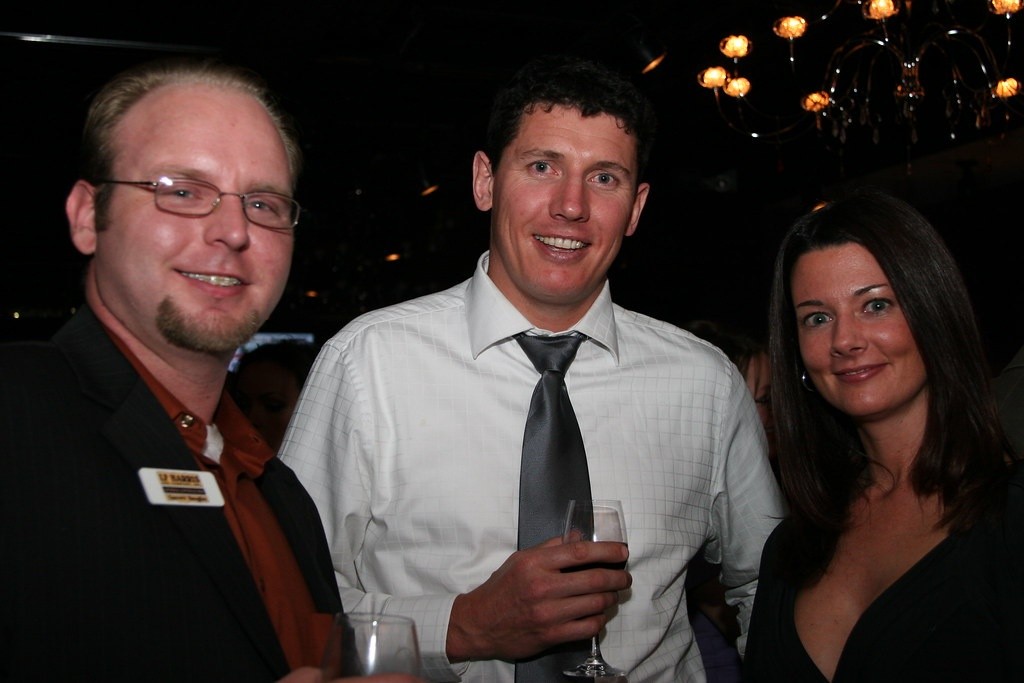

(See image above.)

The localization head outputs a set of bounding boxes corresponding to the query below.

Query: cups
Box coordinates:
[321,613,420,682]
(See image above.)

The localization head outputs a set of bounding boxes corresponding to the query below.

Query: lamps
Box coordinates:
[616,13,666,76]
[695,0,1024,146]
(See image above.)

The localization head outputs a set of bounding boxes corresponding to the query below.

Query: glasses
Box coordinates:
[93,178,300,230]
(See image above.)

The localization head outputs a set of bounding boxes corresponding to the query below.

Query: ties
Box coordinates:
[514,331,595,683]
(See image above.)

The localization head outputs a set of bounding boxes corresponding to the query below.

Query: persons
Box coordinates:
[240,340,320,453]
[740,187,1023,683]
[0,54,424,683]
[990,343,1024,461]
[672,317,782,682]
[277,57,789,683]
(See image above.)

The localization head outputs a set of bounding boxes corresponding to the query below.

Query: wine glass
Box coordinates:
[561,499,627,677]
